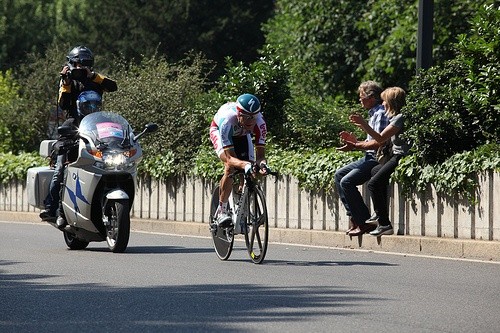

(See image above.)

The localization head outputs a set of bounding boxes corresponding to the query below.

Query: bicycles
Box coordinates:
[208,161,279,263]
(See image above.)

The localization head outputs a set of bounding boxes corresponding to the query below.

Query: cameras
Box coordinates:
[59,60,88,81]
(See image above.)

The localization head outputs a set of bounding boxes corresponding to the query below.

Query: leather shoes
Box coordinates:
[346,225,358,234]
[348,226,365,236]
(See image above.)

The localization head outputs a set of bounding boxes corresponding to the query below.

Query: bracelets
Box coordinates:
[352,142,357,150]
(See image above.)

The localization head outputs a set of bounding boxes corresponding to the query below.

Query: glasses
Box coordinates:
[240,113,259,119]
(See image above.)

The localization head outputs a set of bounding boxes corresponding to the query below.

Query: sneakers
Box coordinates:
[212,209,232,225]
[370,224,393,235]
[365,215,379,224]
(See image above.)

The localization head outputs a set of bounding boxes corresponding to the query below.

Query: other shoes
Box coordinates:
[39,209,57,223]
[56,215,66,227]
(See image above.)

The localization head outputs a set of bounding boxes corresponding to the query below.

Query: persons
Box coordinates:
[334,81,392,235]
[210,94,267,234]
[38,47,117,223]
[349,86,409,237]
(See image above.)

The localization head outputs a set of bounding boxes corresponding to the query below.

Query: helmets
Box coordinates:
[236,93,261,115]
[69,45,94,66]
[76,91,102,117]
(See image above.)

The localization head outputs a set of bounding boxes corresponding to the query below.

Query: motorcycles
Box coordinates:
[26,111,159,254]
